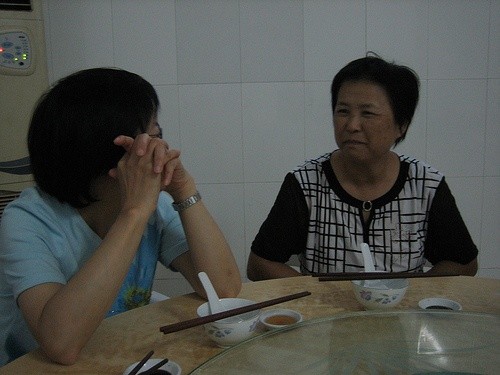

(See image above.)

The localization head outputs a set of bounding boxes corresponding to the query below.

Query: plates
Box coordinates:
[259,308,302,330]
[417,297,462,312]
[123,358,182,375]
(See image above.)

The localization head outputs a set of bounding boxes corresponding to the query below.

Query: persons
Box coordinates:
[0,65,241,368]
[247,51,480,281]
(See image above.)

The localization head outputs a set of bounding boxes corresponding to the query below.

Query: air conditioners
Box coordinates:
[0,0,51,224]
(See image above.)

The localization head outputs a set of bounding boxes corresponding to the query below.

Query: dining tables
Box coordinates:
[0,276,500,375]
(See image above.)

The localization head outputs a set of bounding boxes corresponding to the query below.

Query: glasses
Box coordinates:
[145,127,163,140]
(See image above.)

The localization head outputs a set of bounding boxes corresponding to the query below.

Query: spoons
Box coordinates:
[361,242,387,289]
[198,271,243,323]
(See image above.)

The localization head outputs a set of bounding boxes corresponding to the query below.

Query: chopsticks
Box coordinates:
[311,272,460,282]
[127,350,168,375]
[159,291,312,334]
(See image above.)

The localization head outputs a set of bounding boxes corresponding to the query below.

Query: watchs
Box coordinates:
[171,191,201,212]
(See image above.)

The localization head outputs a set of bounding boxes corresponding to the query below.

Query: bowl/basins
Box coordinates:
[196,298,262,349]
[351,270,408,309]
